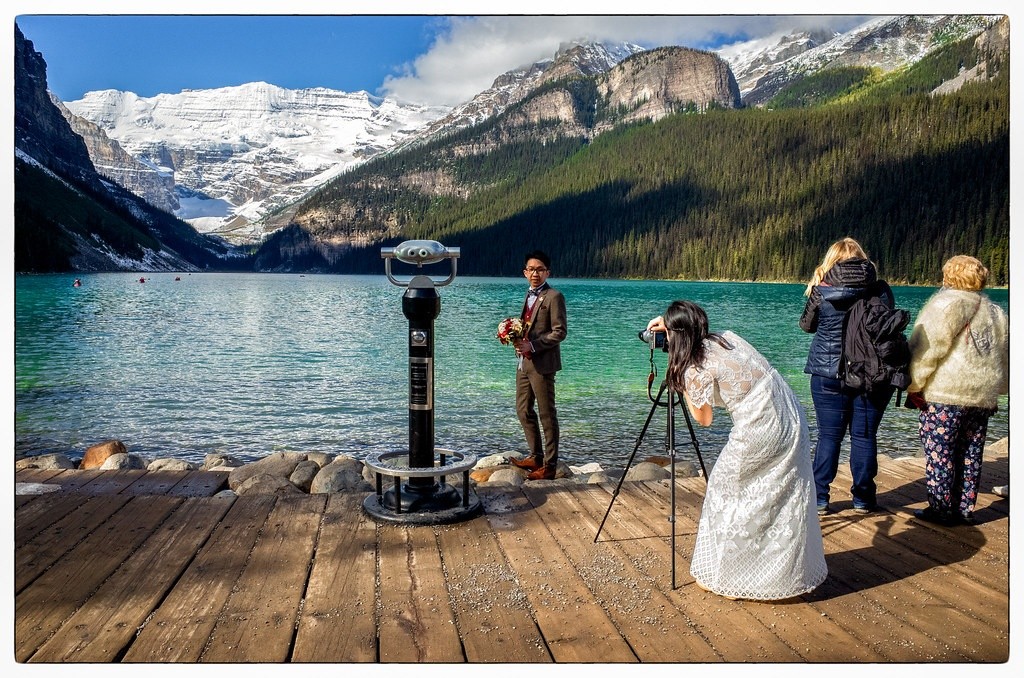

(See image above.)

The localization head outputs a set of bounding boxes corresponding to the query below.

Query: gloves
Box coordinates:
[908,392,929,412]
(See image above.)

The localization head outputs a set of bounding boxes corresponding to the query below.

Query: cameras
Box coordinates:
[638,328,670,353]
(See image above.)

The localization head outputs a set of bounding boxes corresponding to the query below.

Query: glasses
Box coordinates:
[525,267,547,274]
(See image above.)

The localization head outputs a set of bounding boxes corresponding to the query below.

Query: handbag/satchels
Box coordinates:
[904,391,922,410]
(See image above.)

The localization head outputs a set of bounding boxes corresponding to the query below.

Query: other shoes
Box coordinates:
[913,505,958,527]
[852,495,877,514]
[816,492,830,515]
[958,510,975,526]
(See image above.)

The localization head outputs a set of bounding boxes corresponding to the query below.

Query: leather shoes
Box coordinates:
[526,465,556,480]
[510,453,544,471]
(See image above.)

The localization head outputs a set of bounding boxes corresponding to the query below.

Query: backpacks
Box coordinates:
[837,281,913,395]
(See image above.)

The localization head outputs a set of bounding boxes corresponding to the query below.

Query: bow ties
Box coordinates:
[527,285,545,296]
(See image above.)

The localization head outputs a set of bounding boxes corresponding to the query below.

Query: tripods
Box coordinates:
[594,380,709,591]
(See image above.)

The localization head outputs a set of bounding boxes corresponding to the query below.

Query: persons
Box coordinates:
[799,237,896,515]
[905,255,1008,527]
[646,300,829,601]
[508,252,568,481]
